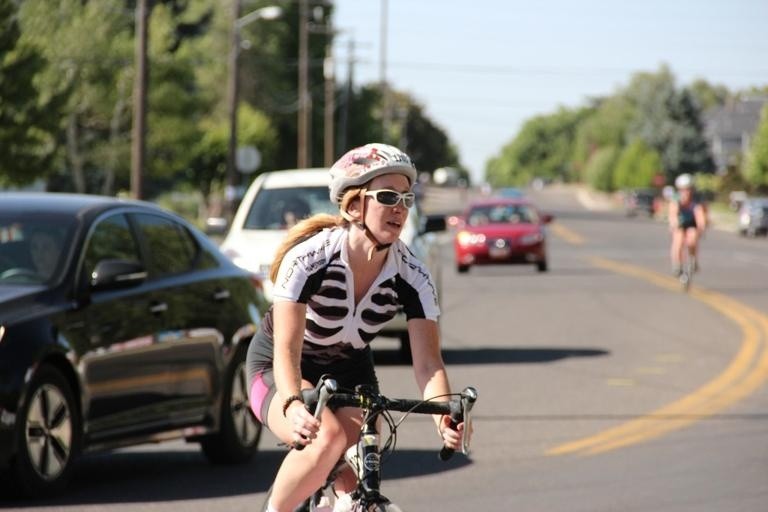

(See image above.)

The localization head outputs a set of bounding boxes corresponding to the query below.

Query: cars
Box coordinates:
[446,196,555,273]
[218,166,448,358]
[738,198,768,236]
[1,190,273,493]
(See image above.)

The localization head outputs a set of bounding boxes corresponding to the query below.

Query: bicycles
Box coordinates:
[261,373,479,512]
[671,222,706,291]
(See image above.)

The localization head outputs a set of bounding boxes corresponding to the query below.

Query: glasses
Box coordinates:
[364,188,416,209]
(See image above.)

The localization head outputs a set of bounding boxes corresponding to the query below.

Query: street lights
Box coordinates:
[224,0,282,186]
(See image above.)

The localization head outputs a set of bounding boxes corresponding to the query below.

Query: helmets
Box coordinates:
[328,141,417,206]
[675,174,694,190]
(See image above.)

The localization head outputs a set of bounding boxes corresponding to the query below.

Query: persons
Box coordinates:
[242,139,475,512]
[510,211,525,221]
[275,199,310,229]
[668,171,708,277]
